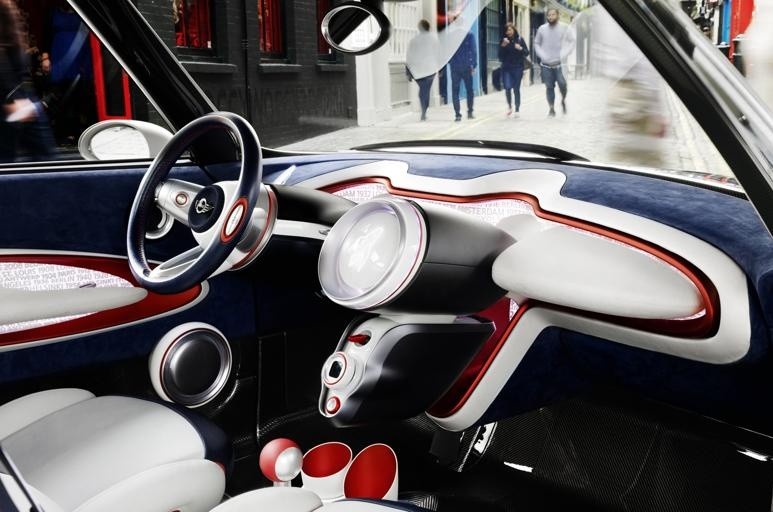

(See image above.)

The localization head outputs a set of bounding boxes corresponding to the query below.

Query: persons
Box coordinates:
[534,7,576,117]
[0,46,58,163]
[406,15,477,121]
[498,23,529,118]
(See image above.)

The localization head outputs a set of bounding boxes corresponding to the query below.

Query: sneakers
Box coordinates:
[418,103,567,122]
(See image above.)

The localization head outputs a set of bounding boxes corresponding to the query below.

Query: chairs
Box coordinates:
[0,388,228,512]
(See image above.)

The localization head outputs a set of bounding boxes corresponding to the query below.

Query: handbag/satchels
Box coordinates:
[522,58,531,69]
